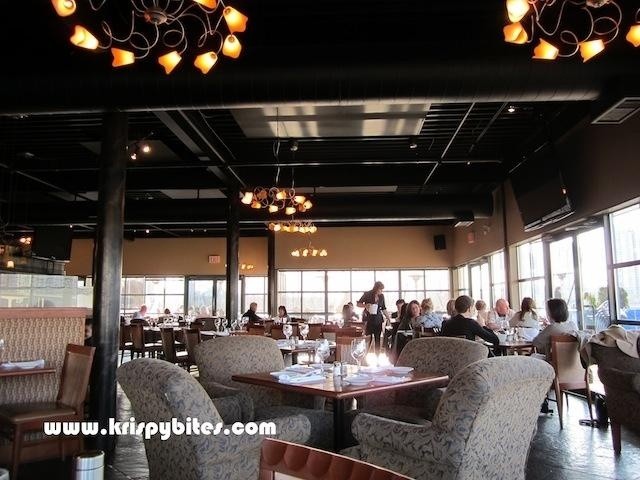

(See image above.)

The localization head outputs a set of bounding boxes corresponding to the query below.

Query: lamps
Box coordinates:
[48,0,252,79]
[126,137,152,161]
[264,142,320,235]
[235,107,315,215]
[625,8,640,50]
[289,232,330,259]
[500,0,622,66]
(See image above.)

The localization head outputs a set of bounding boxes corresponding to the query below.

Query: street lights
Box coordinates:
[409,274,424,298]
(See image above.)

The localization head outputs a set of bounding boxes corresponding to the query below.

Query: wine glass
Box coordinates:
[284,325,293,343]
[213,317,244,334]
[315,338,331,379]
[300,325,309,343]
[351,340,366,376]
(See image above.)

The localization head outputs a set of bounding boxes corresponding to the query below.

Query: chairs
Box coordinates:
[0,307,640,480]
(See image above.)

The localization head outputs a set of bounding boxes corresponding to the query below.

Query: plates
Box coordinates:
[269,372,296,380]
[344,377,374,385]
[388,367,414,374]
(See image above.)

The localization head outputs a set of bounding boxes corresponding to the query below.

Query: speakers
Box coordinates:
[434,234,446,250]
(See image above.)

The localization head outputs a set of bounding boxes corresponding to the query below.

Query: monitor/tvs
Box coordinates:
[507,139,577,232]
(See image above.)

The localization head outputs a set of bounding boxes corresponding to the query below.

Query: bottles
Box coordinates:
[332,361,342,376]
[341,362,348,376]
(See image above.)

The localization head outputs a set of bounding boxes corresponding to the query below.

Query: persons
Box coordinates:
[272,306,290,324]
[133,304,147,320]
[178,304,224,318]
[86,319,93,347]
[241,302,262,327]
[164,308,176,318]
[341,280,581,411]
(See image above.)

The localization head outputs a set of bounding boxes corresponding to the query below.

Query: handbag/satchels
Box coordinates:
[594,393,609,430]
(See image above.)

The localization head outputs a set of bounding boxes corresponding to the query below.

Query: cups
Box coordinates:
[369,304,378,315]
[498,335,507,342]
